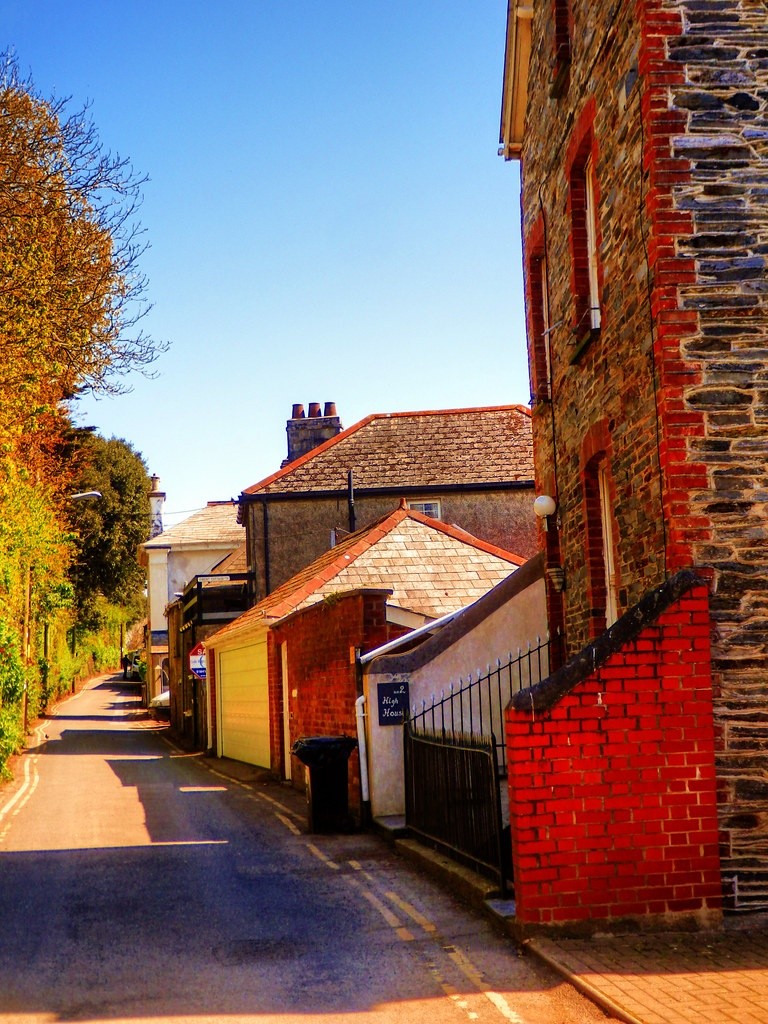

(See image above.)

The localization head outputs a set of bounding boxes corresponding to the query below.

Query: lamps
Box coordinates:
[533,495,561,531]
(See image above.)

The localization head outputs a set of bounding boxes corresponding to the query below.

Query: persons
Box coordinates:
[122,654,132,680]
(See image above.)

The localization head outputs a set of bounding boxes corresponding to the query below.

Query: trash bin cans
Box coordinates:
[292,735,358,834]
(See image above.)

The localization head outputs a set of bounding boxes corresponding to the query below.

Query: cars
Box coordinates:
[130,654,141,677]
[150,689,173,717]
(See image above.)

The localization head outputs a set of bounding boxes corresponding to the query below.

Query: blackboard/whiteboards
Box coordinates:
[377,682,409,726]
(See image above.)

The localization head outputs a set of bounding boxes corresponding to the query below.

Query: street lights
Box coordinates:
[20,492,102,735]
